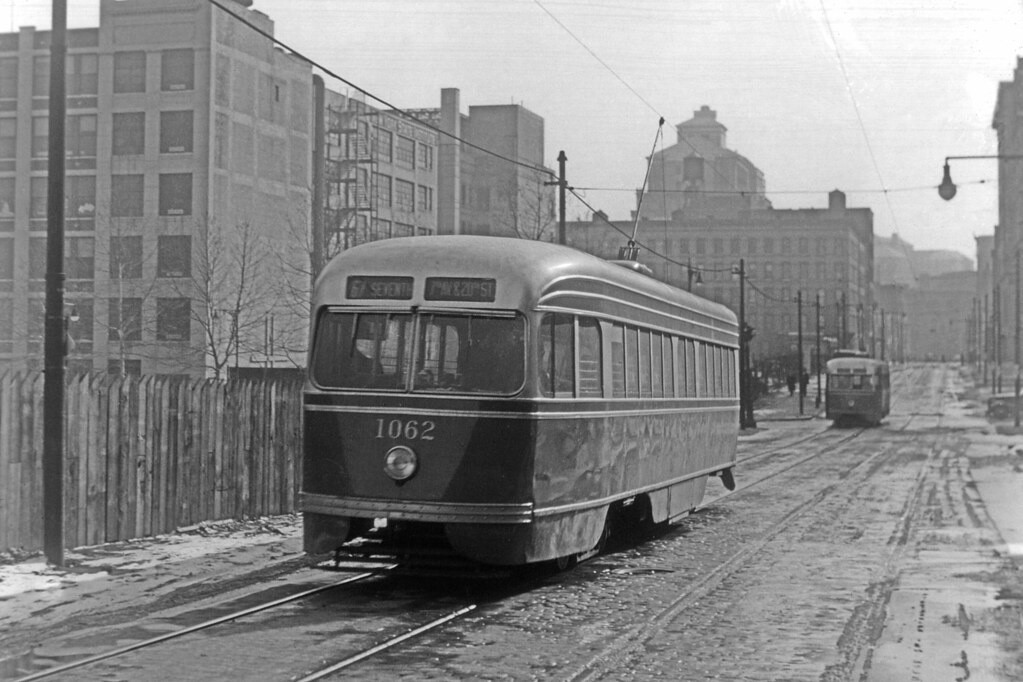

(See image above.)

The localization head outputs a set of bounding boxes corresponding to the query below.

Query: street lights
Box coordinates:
[744,323,756,427]
[688,258,704,292]
[938,155,1023,200]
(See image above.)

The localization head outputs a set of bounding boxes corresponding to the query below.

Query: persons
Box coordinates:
[787,372,797,396]
[801,368,810,397]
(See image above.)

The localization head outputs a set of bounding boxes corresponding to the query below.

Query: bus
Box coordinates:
[294,234,741,577]
[825,357,890,427]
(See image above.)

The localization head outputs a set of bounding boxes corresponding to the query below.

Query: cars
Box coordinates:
[987,389,1023,420]
